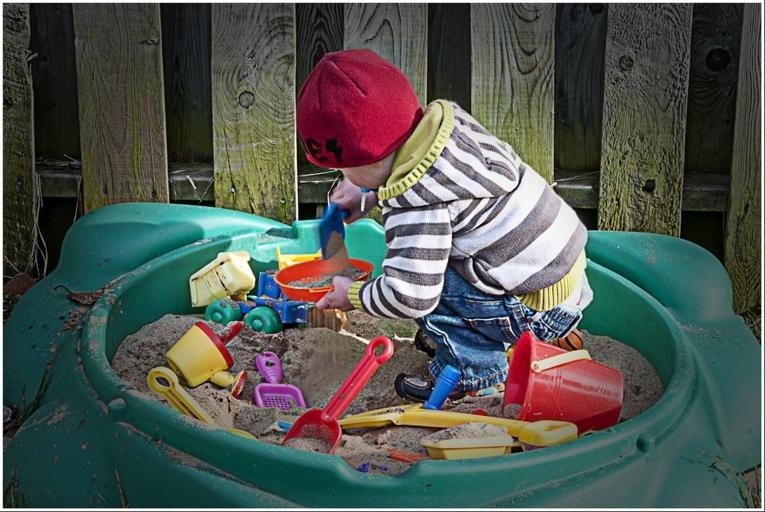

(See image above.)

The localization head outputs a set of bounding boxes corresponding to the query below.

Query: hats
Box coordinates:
[297,48,424,168]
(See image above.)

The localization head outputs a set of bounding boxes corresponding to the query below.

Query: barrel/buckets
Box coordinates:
[164,320,244,386]
[501,329,625,434]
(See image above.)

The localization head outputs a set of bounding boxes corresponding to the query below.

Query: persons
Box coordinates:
[297,47,594,408]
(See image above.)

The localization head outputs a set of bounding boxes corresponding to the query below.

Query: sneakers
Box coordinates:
[416,327,437,358]
[395,370,506,402]
